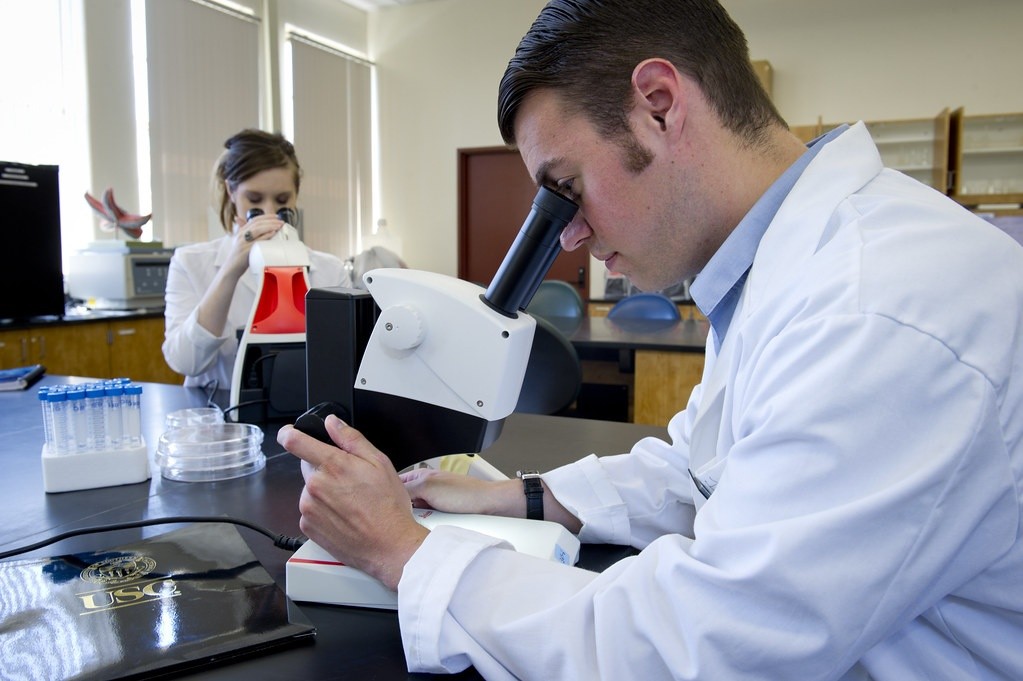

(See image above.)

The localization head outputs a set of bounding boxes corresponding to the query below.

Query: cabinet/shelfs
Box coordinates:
[1,318,186,386]
[787,107,1023,206]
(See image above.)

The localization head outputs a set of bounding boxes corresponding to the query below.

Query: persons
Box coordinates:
[161,128,354,390]
[277,0,1023,681]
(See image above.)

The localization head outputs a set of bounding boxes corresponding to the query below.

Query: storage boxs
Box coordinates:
[287,507,581,609]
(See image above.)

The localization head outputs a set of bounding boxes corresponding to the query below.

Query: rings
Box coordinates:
[245,230,254,241]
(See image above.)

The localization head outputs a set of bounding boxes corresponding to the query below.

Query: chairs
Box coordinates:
[607,293,680,321]
[515,312,583,417]
[525,281,584,318]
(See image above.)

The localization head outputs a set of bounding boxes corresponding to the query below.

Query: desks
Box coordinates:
[538,317,710,426]
[1,374,673,681]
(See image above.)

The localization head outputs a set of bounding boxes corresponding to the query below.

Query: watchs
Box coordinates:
[516,469,545,521]
